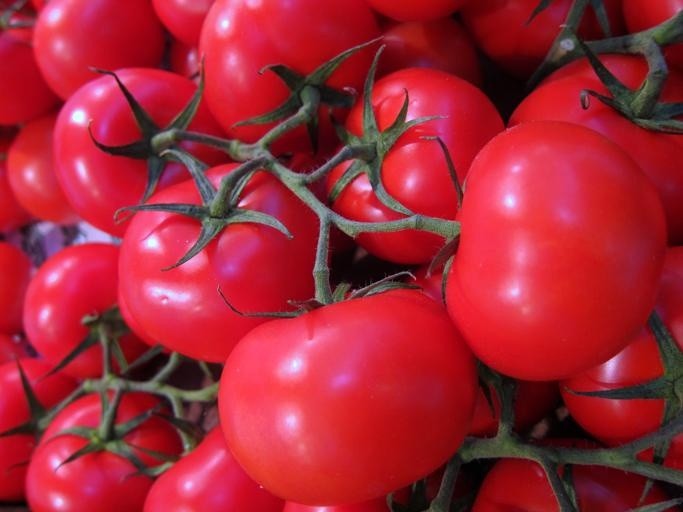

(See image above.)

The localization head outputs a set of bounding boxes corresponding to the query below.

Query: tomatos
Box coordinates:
[0,0,683,512]
